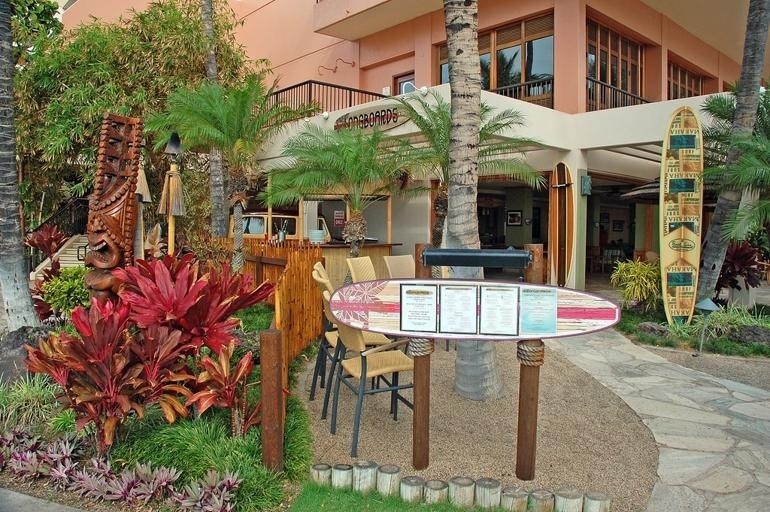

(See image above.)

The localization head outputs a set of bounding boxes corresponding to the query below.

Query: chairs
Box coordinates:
[304,249,416,461]
[591,237,634,279]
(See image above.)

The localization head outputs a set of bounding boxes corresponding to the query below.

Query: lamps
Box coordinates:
[422,248,531,270]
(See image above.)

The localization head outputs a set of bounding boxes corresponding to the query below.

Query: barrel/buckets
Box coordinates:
[308,230,325,245]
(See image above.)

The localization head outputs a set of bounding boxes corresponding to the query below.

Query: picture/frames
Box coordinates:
[506,209,524,227]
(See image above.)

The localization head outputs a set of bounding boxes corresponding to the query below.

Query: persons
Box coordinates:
[599,224,609,264]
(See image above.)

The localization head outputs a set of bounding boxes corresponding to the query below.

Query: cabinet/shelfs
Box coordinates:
[303,237,402,330]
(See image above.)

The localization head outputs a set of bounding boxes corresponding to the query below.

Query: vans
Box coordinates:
[227,212,331,244]
